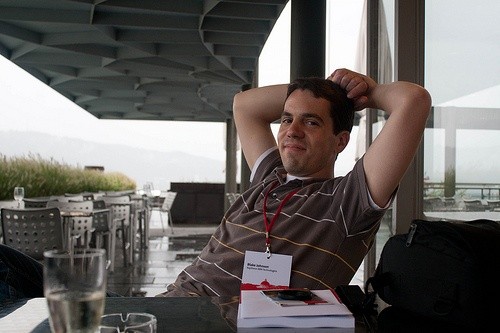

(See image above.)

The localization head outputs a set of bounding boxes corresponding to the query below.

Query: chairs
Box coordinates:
[0,188,178,273]
[0,243,44,298]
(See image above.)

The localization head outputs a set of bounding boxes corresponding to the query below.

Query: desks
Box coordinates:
[0,297,378,333]
[61,211,96,217]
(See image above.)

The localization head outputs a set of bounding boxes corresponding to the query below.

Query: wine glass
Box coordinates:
[14,187,24,208]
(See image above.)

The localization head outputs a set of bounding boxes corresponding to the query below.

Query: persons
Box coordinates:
[154,68,432,297]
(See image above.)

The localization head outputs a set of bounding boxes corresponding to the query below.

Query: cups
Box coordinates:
[44,249,107,333]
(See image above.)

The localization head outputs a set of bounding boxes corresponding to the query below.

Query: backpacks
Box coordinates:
[364,219,500,325]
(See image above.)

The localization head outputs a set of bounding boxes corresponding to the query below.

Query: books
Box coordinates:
[237,288,355,329]
